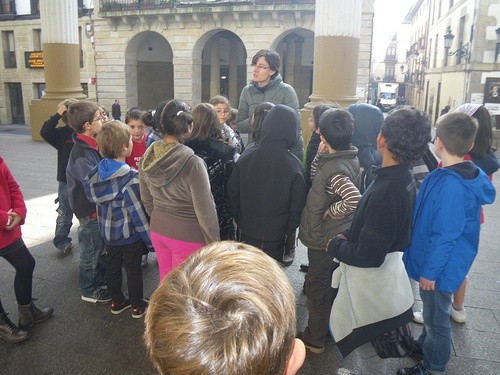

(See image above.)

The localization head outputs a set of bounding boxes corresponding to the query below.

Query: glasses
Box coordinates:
[254,64,271,70]
[93,116,102,122]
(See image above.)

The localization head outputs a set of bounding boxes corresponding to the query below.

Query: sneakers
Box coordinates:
[100,281,108,289]
[132,298,150,318]
[82,289,111,303]
[111,298,131,314]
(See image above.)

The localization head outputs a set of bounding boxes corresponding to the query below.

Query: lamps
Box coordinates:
[400,65,408,75]
[443,28,469,59]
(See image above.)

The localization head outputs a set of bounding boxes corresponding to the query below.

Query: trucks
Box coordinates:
[371,80,401,113]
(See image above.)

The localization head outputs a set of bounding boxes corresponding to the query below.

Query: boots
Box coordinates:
[19,301,53,331]
[283,231,295,265]
[0,309,32,345]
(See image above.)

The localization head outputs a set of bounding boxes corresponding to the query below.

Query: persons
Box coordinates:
[39,48,500,375]
[0,155,53,342]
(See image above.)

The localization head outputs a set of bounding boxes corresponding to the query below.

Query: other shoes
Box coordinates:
[140,254,148,265]
[61,242,73,255]
[296,331,325,354]
[413,310,424,323]
[410,341,424,358]
[450,302,466,323]
[301,264,309,271]
[396,363,433,375]
